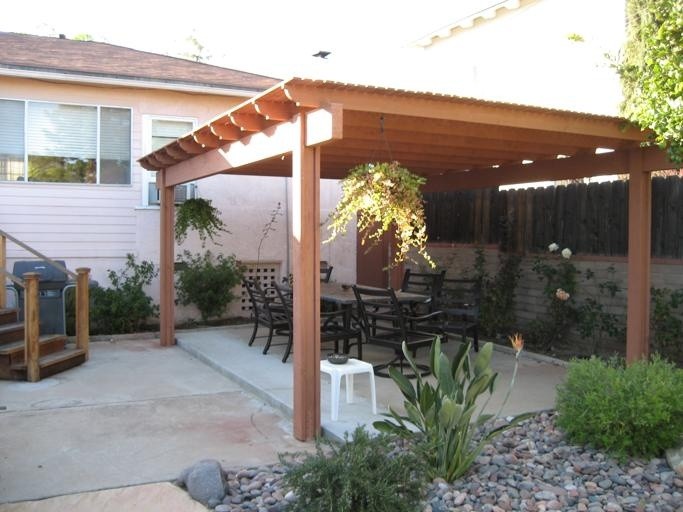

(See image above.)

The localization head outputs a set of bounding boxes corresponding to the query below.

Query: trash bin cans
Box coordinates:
[14,261,68,350]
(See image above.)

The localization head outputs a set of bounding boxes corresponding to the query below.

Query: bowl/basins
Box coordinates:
[326,352,348,364]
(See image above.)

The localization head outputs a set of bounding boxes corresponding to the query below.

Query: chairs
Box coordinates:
[241,260,484,378]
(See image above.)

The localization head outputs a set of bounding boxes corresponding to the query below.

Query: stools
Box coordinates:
[319,358,378,420]
